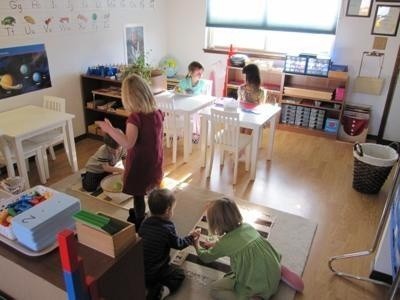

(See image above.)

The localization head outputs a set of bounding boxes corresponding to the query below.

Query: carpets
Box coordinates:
[0,168,319,300]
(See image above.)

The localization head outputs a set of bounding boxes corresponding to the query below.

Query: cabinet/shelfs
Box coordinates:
[80,74,128,141]
[225,61,283,140]
[279,72,350,142]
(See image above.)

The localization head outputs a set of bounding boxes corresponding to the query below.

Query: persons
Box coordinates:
[141,188,202,300]
[81,133,130,195]
[94,75,164,232]
[236,62,266,104]
[176,61,206,143]
[193,197,304,300]
[128,31,144,63]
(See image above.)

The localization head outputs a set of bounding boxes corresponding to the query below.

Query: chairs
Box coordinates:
[152,94,186,163]
[201,78,213,97]
[205,108,252,184]
[0,125,47,187]
[26,95,73,179]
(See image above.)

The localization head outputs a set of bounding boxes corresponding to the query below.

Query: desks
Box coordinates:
[198,100,282,180]
[154,90,217,163]
[0,234,147,300]
[0,105,78,190]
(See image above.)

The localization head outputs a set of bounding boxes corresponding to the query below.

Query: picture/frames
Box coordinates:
[371,5,400,37]
[123,23,147,66]
[345,0,373,18]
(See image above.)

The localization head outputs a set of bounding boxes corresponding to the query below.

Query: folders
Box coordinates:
[359,51,384,79]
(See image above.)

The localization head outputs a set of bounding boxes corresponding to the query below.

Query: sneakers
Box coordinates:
[281,265,304,293]
[158,285,169,299]
